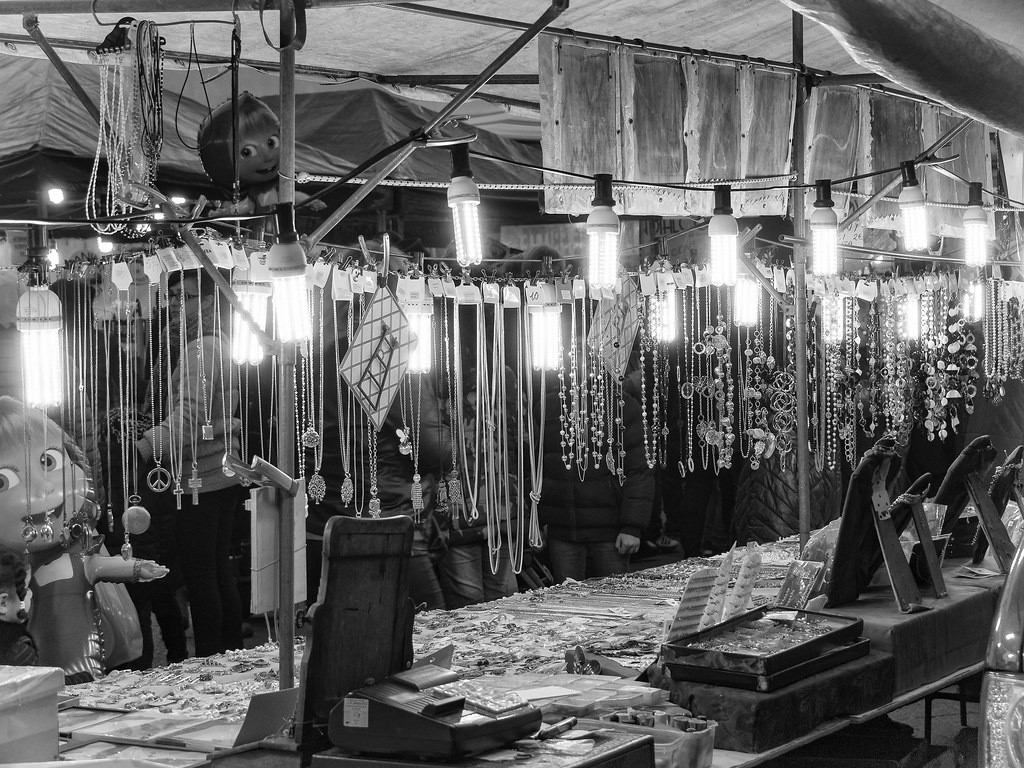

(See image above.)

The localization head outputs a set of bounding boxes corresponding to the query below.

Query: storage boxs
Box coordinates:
[312,552,1009,767]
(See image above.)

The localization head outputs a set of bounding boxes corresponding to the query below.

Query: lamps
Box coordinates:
[446,146,992,281]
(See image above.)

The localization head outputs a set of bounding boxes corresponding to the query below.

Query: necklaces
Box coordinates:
[0,236,1024,575]
[85,21,165,239]
[174,22,214,149]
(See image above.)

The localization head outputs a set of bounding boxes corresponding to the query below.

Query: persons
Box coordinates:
[0,397,167,676]
[198,93,326,237]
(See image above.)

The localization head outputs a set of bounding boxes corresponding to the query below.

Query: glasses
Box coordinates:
[163,290,199,303]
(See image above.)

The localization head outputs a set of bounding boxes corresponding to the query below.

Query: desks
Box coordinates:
[58,517,819,767]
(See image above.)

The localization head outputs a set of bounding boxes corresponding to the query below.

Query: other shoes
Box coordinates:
[647,534,678,550]
